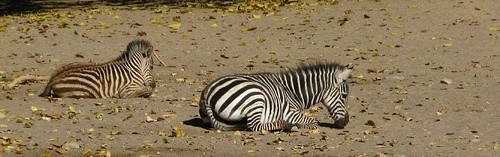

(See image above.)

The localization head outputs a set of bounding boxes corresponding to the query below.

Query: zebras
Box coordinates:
[42,39,156,99]
[199,59,355,133]
